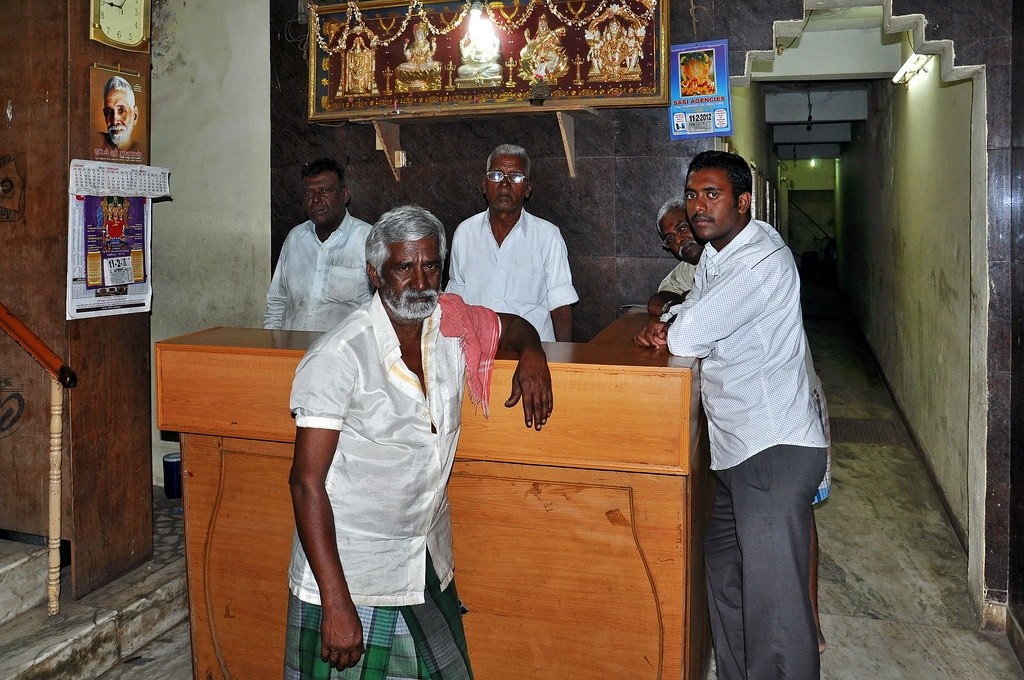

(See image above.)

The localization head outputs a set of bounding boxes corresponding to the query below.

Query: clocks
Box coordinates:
[90,0,151,54]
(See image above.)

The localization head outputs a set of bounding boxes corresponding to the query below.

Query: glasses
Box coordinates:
[662,220,691,249]
[485,171,529,184]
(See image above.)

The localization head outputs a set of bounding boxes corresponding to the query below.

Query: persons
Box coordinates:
[520,15,565,75]
[634,150,828,680]
[94,74,141,155]
[586,20,644,73]
[284,207,547,680]
[346,38,371,91]
[400,23,439,69]
[264,161,373,333]
[649,199,831,653]
[443,144,578,343]
[458,20,503,76]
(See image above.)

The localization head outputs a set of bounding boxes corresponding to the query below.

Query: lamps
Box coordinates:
[892,54,934,84]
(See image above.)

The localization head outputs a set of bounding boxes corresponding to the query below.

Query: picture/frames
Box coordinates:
[308,0,670,114]
